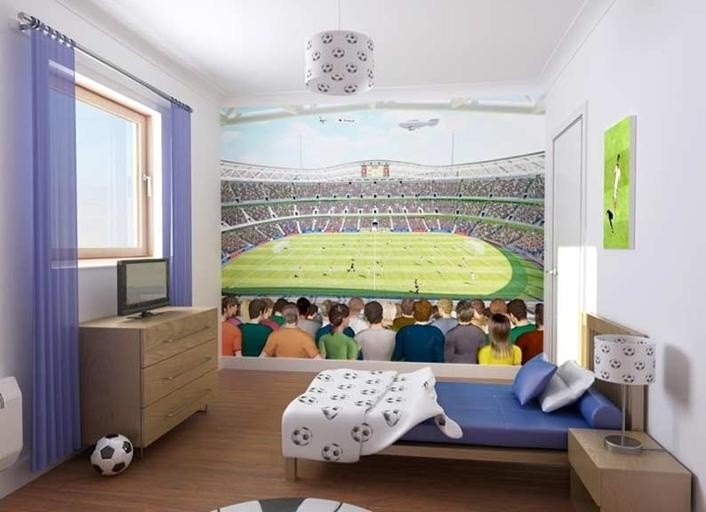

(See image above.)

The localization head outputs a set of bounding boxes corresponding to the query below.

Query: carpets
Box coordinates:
[214,498,375,512]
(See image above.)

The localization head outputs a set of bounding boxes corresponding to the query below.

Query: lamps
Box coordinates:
[304,1,376,97]
[595,334,656,454]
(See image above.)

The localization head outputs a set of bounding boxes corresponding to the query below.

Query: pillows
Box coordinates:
[511,352,557,405]
[540,359,594,412]
[580,389,623,428]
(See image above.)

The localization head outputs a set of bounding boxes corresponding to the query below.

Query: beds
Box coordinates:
[282,310,649,480]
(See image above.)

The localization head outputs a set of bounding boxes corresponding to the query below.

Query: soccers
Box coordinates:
[91,434,134,476]
[593,334,656,385]
[307,30,373,95]
[290,369,445,463]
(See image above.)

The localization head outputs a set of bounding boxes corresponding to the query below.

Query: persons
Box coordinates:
[610,153,620,209]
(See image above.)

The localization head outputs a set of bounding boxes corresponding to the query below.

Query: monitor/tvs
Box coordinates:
[115,256,172,321]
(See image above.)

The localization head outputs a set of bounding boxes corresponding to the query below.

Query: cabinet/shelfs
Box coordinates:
[79,306,219,459]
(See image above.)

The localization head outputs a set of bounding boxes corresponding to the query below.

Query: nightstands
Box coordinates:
[568,428,693,512]
